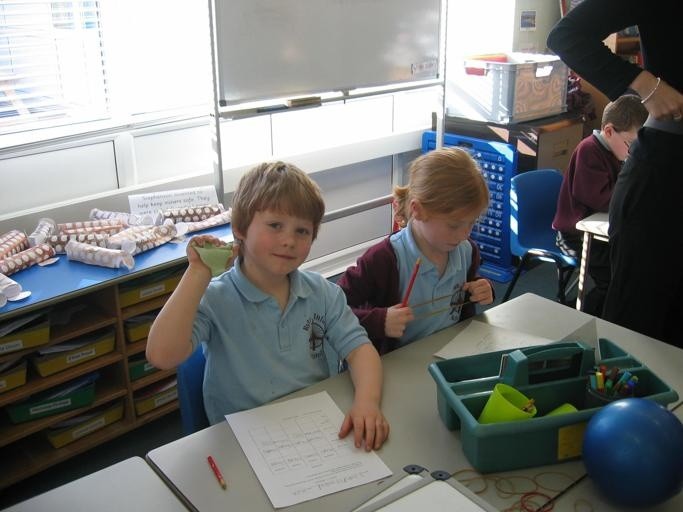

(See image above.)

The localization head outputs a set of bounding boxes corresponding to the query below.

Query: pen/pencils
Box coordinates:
[401,255,423,309]
[586,364,639,400]
[207,456,226,490]
[523,397,536,411]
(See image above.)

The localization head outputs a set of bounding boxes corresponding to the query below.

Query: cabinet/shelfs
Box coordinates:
[0,222,239,490]
[431,107,589,173]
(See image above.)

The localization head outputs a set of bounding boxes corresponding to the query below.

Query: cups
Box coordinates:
[541,401,578,419]
[477,383,538,426]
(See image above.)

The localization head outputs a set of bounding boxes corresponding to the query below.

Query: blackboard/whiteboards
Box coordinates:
[213,0,441,107]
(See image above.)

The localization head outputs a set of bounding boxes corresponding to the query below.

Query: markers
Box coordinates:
[257,106,289,112]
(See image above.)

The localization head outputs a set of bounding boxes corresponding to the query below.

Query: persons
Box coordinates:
[334,145,494,354]
[145,160,389,453]
[549,93,650,318]
[541,1,683,347]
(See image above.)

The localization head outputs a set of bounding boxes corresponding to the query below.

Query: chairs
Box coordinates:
[500,168,609,307]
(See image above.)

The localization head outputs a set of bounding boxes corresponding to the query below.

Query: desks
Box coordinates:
[0,290,683,511]
[572,209,610,312]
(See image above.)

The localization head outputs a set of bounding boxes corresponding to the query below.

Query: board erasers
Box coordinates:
[287,96,321,107]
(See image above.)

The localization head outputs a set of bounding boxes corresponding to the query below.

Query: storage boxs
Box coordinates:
[425,337,680,474]
[445,52,568,126]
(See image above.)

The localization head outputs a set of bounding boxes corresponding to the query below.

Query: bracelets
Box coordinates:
[640,76,661,105]
